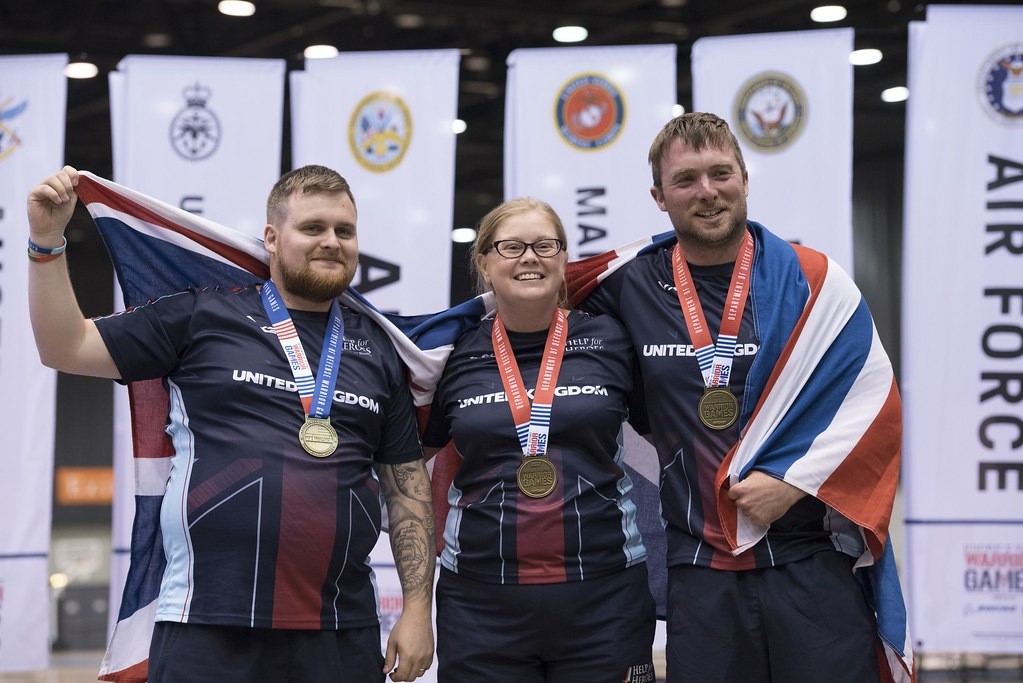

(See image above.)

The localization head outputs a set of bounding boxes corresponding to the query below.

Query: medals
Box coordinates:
[698,386,738,430]
[517,452,558,497]
[298,412,338,458]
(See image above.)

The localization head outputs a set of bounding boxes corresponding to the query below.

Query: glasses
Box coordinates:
[484,239,566,259]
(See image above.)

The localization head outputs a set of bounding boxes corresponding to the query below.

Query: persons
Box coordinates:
[572,110,886,683]
[420,197,657,683]
[24,164,439,683]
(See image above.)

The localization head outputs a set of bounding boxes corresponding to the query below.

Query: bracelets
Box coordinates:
[27,235,67,262]
